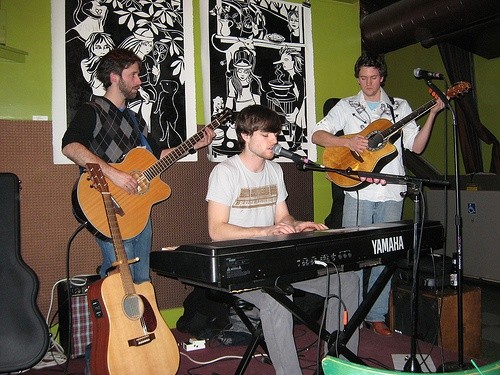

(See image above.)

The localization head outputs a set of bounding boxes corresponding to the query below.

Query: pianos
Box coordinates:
[150,218,444,375]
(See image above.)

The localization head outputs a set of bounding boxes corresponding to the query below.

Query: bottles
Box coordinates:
[450,253,458,290]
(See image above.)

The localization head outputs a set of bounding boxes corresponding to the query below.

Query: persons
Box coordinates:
[310,55,450,337]
[61,48,215,285]
[205,104,360,375]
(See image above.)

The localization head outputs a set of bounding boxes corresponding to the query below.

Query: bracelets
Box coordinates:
[188,147,196,154]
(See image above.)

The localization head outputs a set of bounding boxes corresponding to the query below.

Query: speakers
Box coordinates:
[56,275,103,359]
[423,174,500,284]
[390,283,482,357]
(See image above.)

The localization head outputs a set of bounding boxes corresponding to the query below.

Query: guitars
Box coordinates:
[83,163,180,375]
[71,107,236,241]
[322,80,474,190]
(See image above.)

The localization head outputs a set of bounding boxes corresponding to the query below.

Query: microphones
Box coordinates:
[413,67,444,80]
[274,145,314,165]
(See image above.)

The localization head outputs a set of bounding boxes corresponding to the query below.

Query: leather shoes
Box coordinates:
[371,321,391,337]
